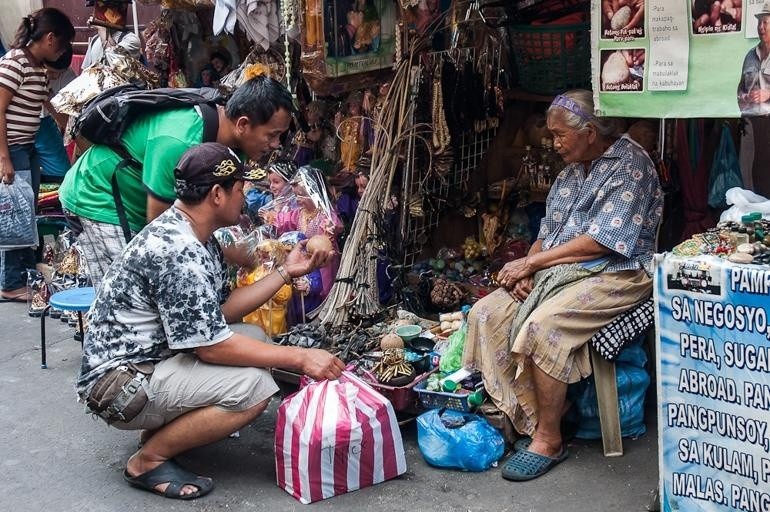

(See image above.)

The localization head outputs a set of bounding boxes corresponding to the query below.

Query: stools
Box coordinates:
[587,297,657,457]
[40,286,97,368]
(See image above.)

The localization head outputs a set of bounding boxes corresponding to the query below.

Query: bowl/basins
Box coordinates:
[408,337,436,355]
[396,325,422,344]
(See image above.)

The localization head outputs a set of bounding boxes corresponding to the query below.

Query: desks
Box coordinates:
[653,225,770,512]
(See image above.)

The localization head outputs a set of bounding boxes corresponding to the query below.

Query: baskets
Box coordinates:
[365,345,438,413]
[413,379,485,413]
[508,24,591,96]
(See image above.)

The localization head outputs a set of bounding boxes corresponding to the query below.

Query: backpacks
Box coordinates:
[69,84,226,171]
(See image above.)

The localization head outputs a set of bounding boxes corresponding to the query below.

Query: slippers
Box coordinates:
[514,436,532,452]
[502,448,569,481]
[0,287,36,302]
[123,457,214,500]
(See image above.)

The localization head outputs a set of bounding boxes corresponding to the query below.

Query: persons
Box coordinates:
[601,49,645,92]
[277,79,399,167]
[212,162,374,347]
[142,2,182,71]
[77,142,347,500]
[601,0,645,37]
[691,0,741,35]
[0,7,75,304]
[736,0,770,118]
[460,89,668,483]
[34,43,78,176]
[57,73,294,297]
[79,0,148,71]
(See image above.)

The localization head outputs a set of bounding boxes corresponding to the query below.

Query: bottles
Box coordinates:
[434,380,456,406]
[456,393,482,408]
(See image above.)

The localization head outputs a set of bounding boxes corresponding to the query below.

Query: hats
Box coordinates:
[174,142,267,181]
[754,0,770,18]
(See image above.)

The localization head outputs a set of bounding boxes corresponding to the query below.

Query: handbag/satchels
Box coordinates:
[0,170,39,252]
[86,361,155,426]
